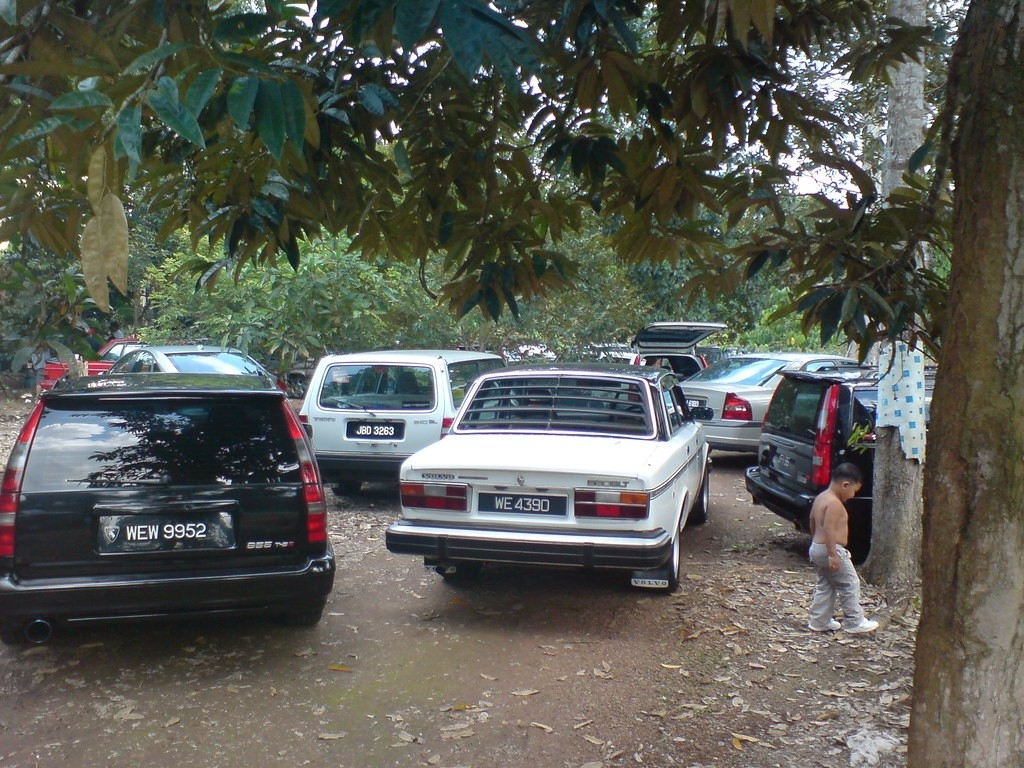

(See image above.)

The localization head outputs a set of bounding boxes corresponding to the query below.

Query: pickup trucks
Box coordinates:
[39,339,192,393]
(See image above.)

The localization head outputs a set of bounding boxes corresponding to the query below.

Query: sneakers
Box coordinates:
[808,618,842,632]
[845,619,879,633]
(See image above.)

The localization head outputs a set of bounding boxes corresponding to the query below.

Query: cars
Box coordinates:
[385,361,714,592]
[99,345,285,394]
[680,352,850,454]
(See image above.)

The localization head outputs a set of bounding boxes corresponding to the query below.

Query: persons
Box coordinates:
[31,344,50,388]
[808,463,879,633]
[106,322,124,342]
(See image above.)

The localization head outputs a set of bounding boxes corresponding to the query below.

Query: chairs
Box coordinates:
[508,389,560,429]
[391,373,420,396]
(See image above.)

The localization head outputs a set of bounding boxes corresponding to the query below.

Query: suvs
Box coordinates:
[744,358,937,556]
[298,348,506,497]
[585,321,729,385]
[0,373,337,648]
